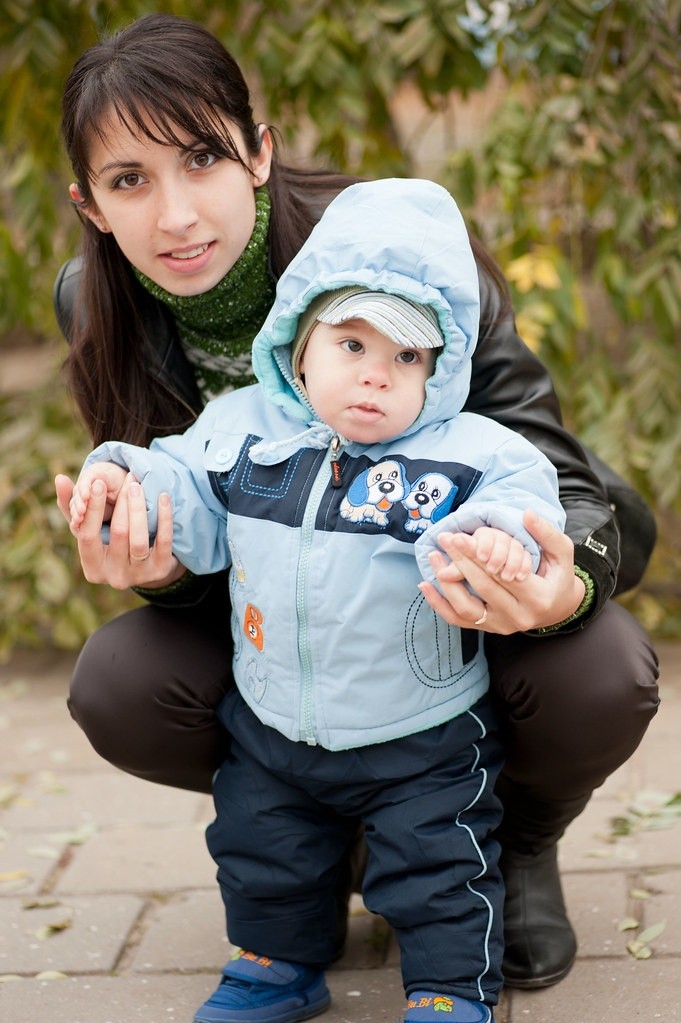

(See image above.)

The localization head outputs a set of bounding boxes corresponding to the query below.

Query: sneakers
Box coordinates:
[190,949,333,1022]
[404,988,495,1023]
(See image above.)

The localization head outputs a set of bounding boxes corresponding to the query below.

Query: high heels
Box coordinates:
[499,841,577,991]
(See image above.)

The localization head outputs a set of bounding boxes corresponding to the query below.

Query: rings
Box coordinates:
[130,552,150,560]
[475,607,488,626]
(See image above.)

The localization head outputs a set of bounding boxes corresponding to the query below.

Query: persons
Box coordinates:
[50,177,561,1023]
[56,22,660,992]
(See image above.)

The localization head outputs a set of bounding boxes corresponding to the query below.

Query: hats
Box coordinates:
[290,286,444,389]
